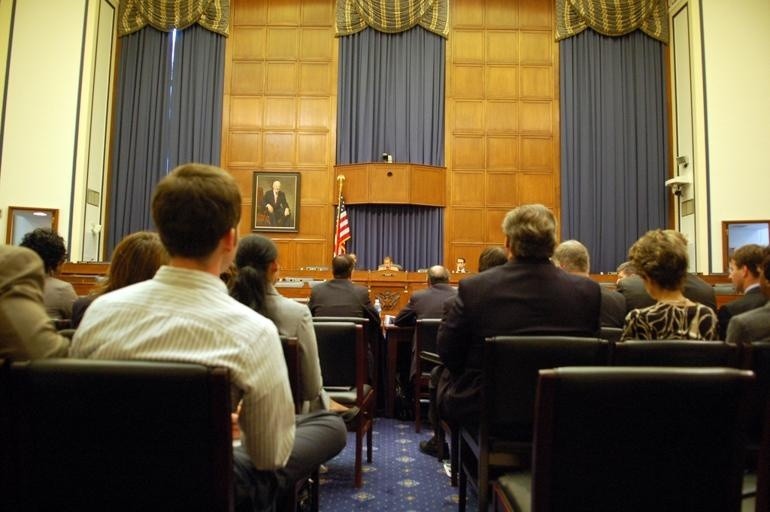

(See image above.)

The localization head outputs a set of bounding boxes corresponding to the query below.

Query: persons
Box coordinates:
[378,256,398,271]
[308,254,383,417]
[616,262,637,284]
[420,248,508,459]
[74,160,349,491]
[0,243,72,361]
[434,206,601,427]
[451,257,470,273]
[393,266,457,421]
[716,244,770,343]
[557,240,626,328]
[619,228,721,342]
[261,179,291,228]
[21,199,360,472]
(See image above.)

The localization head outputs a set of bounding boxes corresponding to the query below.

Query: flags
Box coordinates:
[331,185,352,260]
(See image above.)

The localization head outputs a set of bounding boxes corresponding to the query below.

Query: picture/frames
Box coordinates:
[250,170,302,234]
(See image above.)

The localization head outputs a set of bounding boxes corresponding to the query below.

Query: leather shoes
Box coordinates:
[419,439,449,459]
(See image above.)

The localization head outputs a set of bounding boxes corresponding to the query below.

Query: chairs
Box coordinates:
[377,264,405,271]
[411,317,770,511]
[2,318,382,511]
